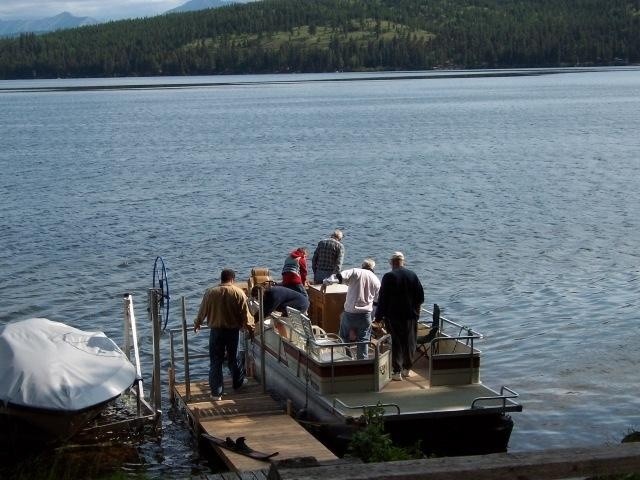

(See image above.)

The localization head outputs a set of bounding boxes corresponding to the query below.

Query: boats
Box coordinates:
[229,258,526,455]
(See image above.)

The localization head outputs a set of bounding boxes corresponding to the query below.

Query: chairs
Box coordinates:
[307,337,355,365]
[408,301,444,368]
[285,305,329,348]
[298,313,348,356]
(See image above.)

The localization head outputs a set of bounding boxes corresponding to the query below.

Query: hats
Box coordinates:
[390,252,404,260]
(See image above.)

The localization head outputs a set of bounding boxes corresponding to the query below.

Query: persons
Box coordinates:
[372,251,424,381]
[312,230,345,285]
[282,247,309,297]
[251,283,311,325]
[193,269,255,401]
[320,259,381,360]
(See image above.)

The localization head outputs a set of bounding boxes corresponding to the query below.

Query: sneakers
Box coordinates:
[402,368,408,377]
[236,378,248,391]
[392,373,402,380]
[210,393,222,401]
